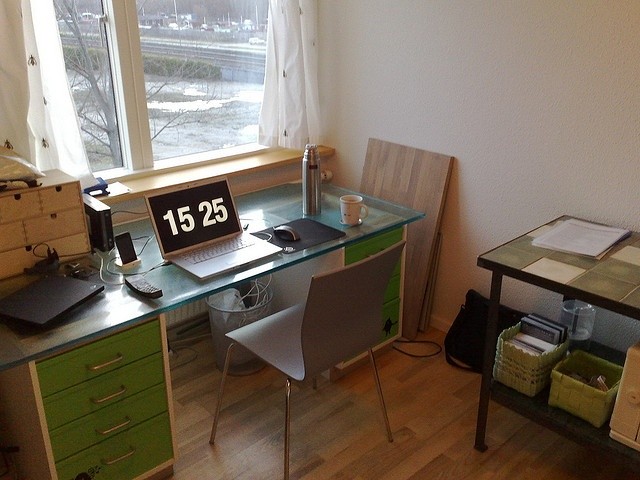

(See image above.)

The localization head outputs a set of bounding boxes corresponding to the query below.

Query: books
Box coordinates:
[502,311,569,358]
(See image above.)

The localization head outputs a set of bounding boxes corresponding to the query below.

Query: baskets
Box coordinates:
[492,321,570,398]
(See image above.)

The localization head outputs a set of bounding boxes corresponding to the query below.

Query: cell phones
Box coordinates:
[115,232,138,265]
[64,259,94,279]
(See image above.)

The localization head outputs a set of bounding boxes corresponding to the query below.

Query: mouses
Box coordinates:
[274,224,301,242]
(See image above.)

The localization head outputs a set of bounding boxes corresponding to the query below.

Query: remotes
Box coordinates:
[125,273,164,300]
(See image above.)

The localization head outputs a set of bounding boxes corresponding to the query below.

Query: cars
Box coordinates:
[249,37,265,45]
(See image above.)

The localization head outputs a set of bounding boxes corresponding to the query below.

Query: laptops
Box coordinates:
[142,173,283,282]
[0,273,104,332]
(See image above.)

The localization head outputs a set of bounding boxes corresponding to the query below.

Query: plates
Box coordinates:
[340,219,363,226]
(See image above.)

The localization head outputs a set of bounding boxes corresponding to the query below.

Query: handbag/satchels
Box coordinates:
[444,289,529,374]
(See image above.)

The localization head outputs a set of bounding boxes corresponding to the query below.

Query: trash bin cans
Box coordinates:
[205,281,274,376]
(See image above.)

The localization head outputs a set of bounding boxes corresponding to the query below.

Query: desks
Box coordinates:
[474,212,639,473]
[0,185,428,480]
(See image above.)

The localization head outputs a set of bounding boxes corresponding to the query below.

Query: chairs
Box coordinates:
[210,242,406,480]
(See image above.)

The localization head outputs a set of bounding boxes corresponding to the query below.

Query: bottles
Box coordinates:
[301,144,321,216]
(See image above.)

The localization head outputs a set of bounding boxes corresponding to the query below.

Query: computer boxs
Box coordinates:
[82,193,116,261]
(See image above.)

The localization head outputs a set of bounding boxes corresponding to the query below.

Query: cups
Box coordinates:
[340,195,368,224]
